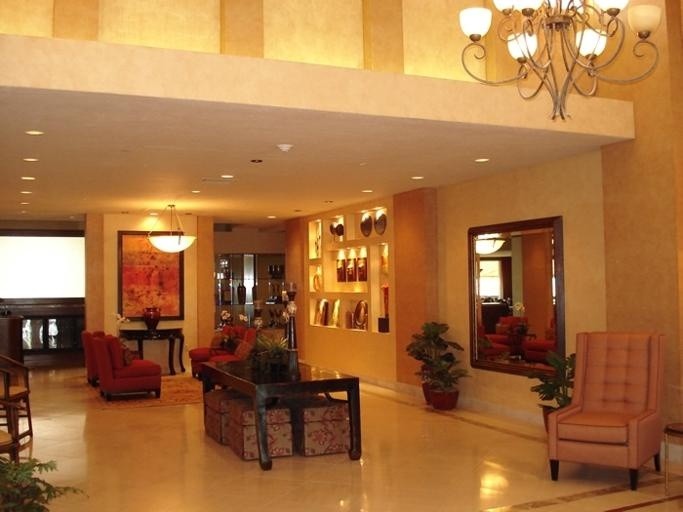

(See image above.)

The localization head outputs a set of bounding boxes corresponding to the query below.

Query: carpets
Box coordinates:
[79,366,203,409]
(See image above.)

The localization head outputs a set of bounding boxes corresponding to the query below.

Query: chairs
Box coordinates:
[0,353,34,465]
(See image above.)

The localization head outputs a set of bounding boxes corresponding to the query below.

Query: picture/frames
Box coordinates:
[116,229,185,321]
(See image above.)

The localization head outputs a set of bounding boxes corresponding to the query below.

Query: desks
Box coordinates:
[197,359,362,471]
[118,328,186,375]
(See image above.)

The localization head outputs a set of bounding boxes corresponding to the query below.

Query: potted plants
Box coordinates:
[531,350,577,434]
[250,333,302,385]
[406,321,473,411]
[0,455,89,512]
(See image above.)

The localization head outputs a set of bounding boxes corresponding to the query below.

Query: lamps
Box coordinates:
[148,203,195,254]
[459,0,661,123]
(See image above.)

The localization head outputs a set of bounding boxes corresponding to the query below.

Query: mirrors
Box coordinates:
[284,281,301,382]
[214,251,287,332]
[466,216,566,382]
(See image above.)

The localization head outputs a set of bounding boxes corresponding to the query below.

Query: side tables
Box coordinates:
[663,421,683,491]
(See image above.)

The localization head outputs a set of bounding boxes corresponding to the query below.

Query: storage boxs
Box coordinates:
[203,387,351,461]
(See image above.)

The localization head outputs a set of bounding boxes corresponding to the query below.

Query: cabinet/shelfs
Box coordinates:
[302,187,441,388]
[216,253,284,305]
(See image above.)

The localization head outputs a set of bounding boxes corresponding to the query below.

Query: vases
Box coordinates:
[142,308,162,329]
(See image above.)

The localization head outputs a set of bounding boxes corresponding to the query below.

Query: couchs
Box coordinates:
[188,325,255,379]
[82,330,161,404]
[477,315,528,356]
[546,329,668,491]
[522,339,555,362]
